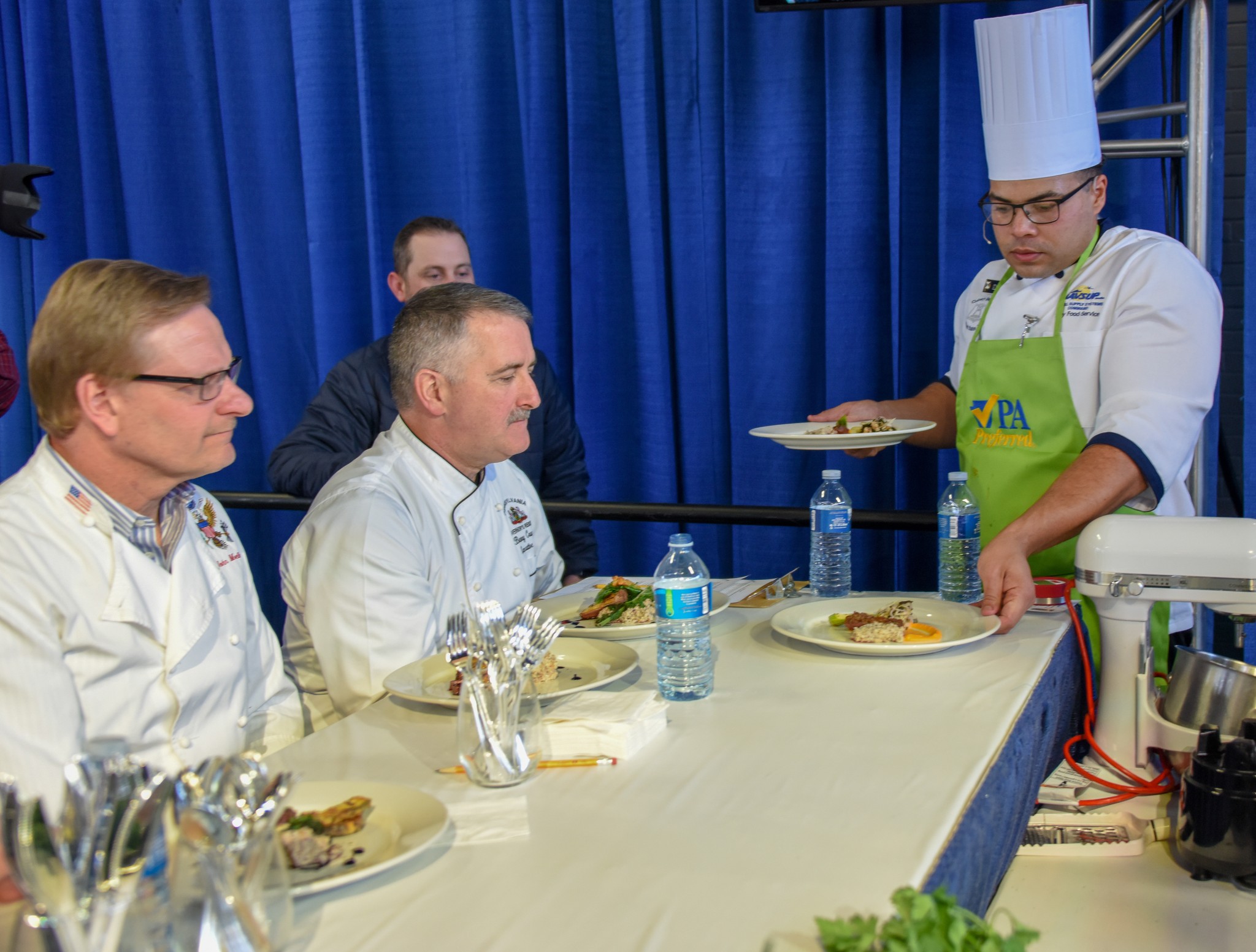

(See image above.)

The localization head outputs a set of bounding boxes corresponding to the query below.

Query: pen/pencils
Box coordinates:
[435,756,617,774]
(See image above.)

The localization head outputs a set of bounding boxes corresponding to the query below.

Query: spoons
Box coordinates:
[173,751,294,952]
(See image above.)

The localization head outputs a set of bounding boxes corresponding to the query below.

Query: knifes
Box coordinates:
[0,752,174,952]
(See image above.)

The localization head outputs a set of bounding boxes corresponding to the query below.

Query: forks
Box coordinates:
[444,600,564,781]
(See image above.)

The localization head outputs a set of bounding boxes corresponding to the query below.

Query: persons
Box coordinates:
[807,5,1223,704]
[0,260,306,824]
[277,281,566,735]
[267,215,597,588]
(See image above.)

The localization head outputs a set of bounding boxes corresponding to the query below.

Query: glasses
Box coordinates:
[95,356,243,402]
[976,175,1098,226]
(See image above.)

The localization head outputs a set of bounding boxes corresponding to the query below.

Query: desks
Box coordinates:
[266,575,1093,952]
[985,758,1256,952]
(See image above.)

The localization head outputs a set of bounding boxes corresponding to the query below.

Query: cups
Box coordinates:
[12,892,154,952]
[166,832,290,952]
[456,669,545,788]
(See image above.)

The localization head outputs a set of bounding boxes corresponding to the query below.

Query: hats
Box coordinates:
[973,1,1101,181]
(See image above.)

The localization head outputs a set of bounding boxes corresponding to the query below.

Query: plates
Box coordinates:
[749,419,936,450]
[282,779,451,898]
[769,597,1001,657]
[516,588,730,642]
[382,637,638,710]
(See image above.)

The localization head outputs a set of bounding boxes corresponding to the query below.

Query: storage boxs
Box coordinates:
[1016,813,1150,856]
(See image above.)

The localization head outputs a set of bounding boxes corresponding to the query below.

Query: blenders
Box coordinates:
[1075,512,1256,817]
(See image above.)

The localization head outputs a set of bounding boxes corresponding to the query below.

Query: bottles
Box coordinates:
[809,470,852,598]
[653,533,714,701]
[83,737,176,952]
[937,471,982,604]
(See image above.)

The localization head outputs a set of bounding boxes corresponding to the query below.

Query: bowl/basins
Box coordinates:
[1162,645,1256,737]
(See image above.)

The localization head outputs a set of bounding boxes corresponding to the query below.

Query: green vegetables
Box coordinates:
[592,580,655,627]
[835,415,847,427]
[286,814,325,835]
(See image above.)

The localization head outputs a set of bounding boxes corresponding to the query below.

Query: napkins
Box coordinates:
[426,796,530,846]
[527,689,671,760]
